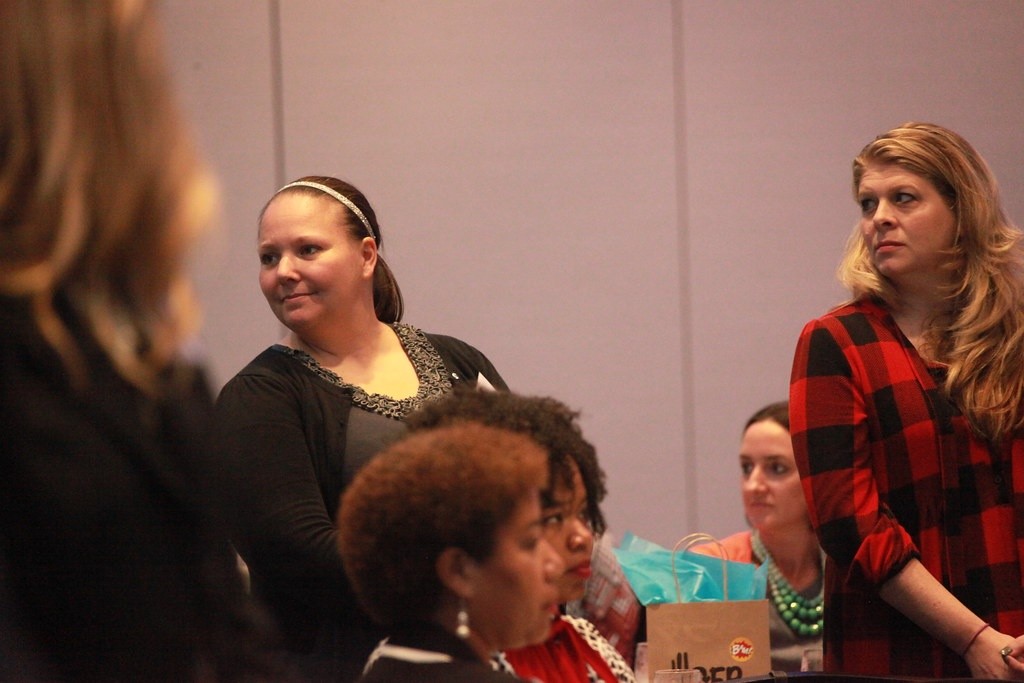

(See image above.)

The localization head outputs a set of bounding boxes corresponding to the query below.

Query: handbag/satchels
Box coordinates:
[645,533,774,683]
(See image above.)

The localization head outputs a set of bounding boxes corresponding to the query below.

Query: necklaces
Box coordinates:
[750,533,825,637]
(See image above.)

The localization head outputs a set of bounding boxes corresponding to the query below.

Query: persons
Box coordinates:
[0,0,298,683]
[789,124,1024,683]
[684,400,826,672]
[215,176,511,683]
[340,391,636,683]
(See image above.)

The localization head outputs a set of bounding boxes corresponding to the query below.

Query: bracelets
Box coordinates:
[962,623,990,659]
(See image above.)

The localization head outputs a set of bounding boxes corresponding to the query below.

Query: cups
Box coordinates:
[654,670,702,683]
[634,642,648,683]
[800,649,822,672]
